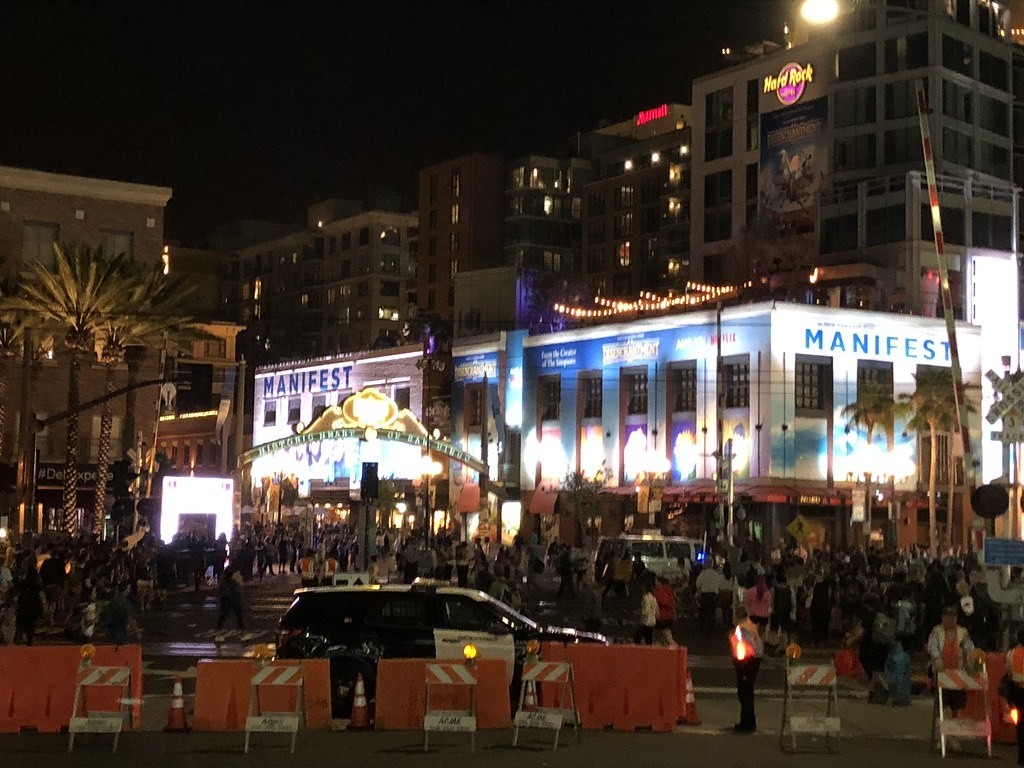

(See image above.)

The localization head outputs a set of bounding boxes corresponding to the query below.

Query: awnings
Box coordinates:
[605,481,747,503]
[743,486,798,504]
[530,483,560,515]
[457,483,480,512]
[786,484,937,509]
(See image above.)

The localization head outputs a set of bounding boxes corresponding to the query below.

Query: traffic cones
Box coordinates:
[525,679,538,709]
[684,672,701,726]
[166,677,188,732]
[347,672,372,730]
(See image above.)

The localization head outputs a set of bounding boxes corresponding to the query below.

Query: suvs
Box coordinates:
[275,576,611,719]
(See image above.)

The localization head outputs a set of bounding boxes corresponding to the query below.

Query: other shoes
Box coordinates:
[215,635,225,642]
[241,633,254,642]
[734,720,757,733]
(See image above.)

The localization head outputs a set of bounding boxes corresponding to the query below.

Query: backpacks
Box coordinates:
[872,614,898,645]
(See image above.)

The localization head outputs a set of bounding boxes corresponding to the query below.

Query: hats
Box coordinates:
[942,606,957,616]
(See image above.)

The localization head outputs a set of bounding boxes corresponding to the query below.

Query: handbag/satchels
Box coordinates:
[834,650,863,677]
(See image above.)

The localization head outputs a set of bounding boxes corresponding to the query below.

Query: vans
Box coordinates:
[594,536,707,595]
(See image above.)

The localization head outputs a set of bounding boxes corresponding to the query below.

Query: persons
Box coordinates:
[728,607,763,732]
[1006,630,1024,692]
[216,557,248,629]
[927,607,975,718]
[374,328,397,350]
[0,533,228,646]
[527,531,592,594]
[229,521,304,582]
[693,528,1024,686]
[301,520,358,586]
[512,530,525,570]
[468,537,531,617]
[434,525,469,587]
[374,522,438,580]
[599,540,678,647]
